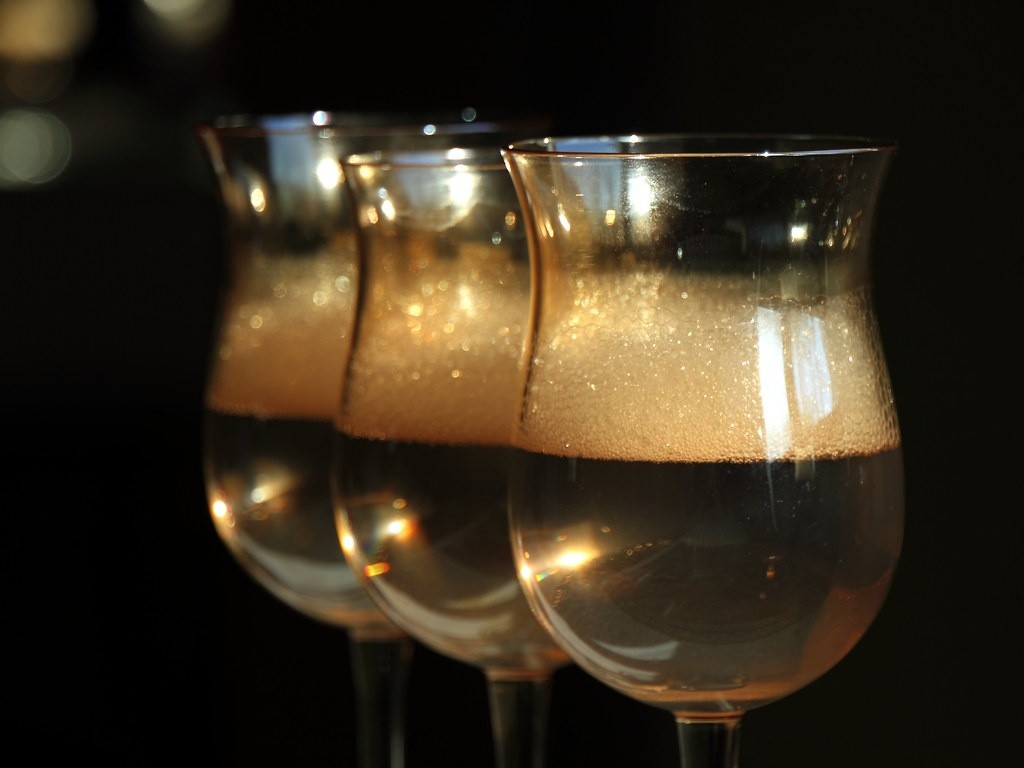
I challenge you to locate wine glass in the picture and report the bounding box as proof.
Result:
[499,133,905,768]
[195,113,549,768]
[334,148,572,768]
